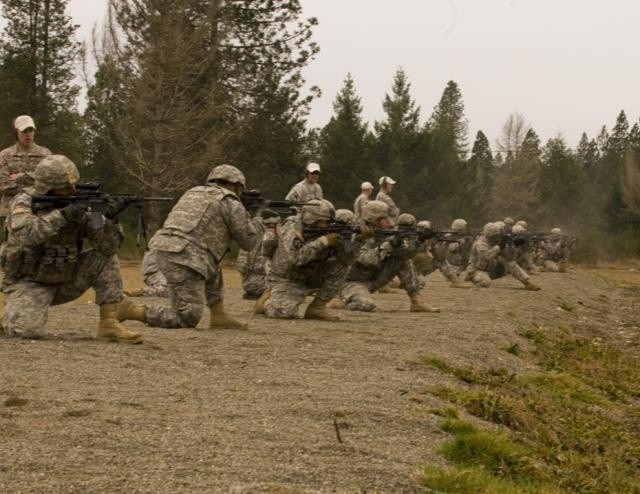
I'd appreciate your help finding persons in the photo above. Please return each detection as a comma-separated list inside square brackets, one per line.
[124, 246, 173, 297]
[375, 175, 401, 220]
[241, 199, 467, 320]
[284, 161, 324, 210]
[443, 217, 570, 290]
[0, 113, 54, 237]
[0, 154, 143, 345]
[119, 163, 266, 329]
[354, 181, 375, 217]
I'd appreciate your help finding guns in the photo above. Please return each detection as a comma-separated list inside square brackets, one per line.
[237, 189, 321, 219]
[399, 223, 584, 246]
[33, 182, 175, 219]
[303, 217, 407, 254]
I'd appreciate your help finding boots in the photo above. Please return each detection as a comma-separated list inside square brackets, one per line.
[524, 278, 541, 290]
[411, 291, 440, 312]
[97, 301, 143, 343]
[254, 288, 271, 314]
[124, 290, 145, 297]
[450, 275, 473, 288]
[117, 294, 146, 321]
[304, 296, 339, 321]
[379, 283, 397, 293]
[209, 300, 247, 330]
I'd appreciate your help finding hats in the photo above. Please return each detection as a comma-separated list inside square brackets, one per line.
[14, 114, 37, 131]
[361, 181, 374, 191]
[306, 163, 321, 173]
[379, 176, 396, 185]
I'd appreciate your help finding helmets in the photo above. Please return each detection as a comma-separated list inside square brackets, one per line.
[482, 217, 528, 236]
[550, 227, 562, 235]
[302, 197, 433, 234]
[450, 218, 468, 233]
[33, 154, 80, 194]
[206, 164, 245, 193]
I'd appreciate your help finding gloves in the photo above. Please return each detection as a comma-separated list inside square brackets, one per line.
[60, 203, 88, 228]
[355, 224, 373, 241]
[513, 237, 524, 246]
[105, 195, 125, 218]
[418, 232, 434, 242]
[324, 232, 342, 246]
[498, 238, 507, 250]
[390, 236, 403, 247]
